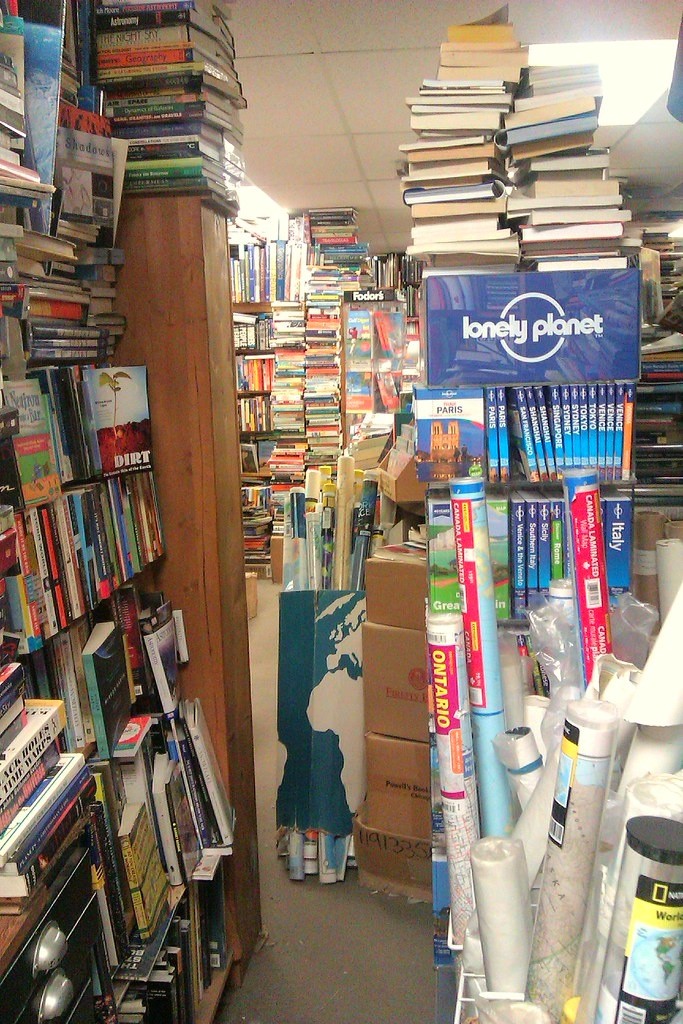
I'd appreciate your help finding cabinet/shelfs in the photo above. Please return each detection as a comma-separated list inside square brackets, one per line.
[110, 186, 263, 991]
[0, 847, 103, 1024]
[421, 478, 640, 625]
[232, 298, 345, 479]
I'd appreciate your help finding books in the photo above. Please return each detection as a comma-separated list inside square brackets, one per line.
[0, 3, 247, 1024]
[227, 2, 683, 625]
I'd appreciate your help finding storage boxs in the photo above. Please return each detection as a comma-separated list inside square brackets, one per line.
[352, 816, 433, 901]
[361, 621, 429, 742]
[356, 730, 434, 840]
[365, 557, 428, 630]
[270, 534, 284, 585]
[243, 572, 259, 620]
